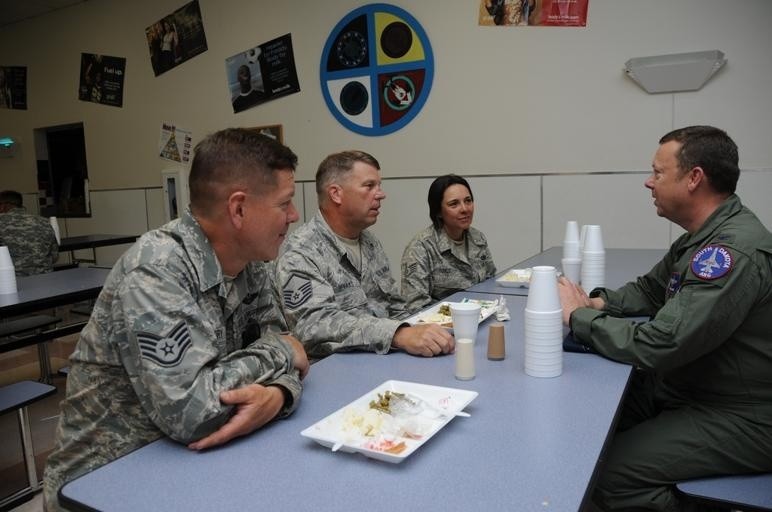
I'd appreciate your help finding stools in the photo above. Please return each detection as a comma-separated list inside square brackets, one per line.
[670, 472, 772, 511]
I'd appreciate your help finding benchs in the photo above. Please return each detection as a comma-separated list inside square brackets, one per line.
[1, 362, 69, 512]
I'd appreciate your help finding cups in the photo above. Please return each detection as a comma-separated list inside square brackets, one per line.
[449, 299, 482, 346]
[0, 244, 18, 295]
[560, 221, 607, 296]
[48, 216, 62, 246]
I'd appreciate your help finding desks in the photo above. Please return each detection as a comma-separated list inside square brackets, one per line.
[2, 229, 143, 384]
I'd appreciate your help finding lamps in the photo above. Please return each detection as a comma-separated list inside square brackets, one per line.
[623, 50, 728, 94]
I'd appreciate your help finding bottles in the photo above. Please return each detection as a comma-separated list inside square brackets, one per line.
[486, 324, 507, 361]
[523, 264, 564, 379]
[454, 336, 476, 381]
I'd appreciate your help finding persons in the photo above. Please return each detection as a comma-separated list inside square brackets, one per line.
[268, 150, 455, 366]
[400, 175, 496, 314]
[0, 191, 59, 277]
[558, 126, 772, 512]
[42, 129, 309, 512]
[160, 21, 179, 69]
[232, 65, 264, 113]
